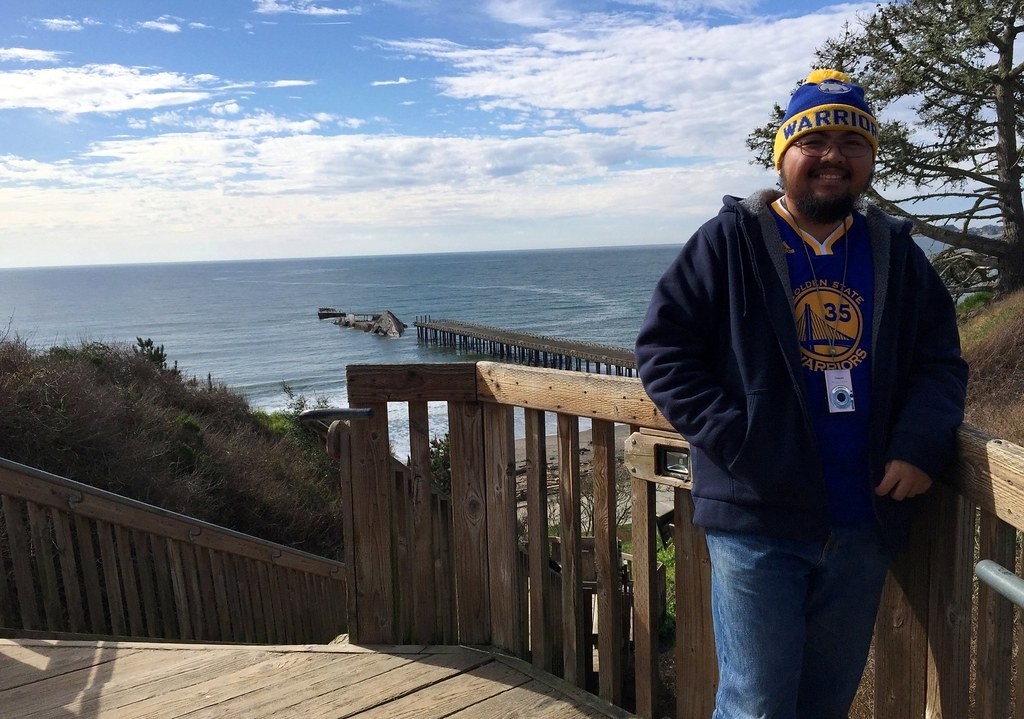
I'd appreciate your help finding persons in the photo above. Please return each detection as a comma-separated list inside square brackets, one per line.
[635, 67, 970, 719]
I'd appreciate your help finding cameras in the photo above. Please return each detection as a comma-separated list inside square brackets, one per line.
[824, 370, 855, 413]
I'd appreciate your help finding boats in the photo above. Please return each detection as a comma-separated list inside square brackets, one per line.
[317, 306, 347, 319]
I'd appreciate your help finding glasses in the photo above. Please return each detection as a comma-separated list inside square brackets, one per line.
[793, 132, 874, 159]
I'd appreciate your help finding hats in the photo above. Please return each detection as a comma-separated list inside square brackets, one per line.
[774, 67, 878, 172]
